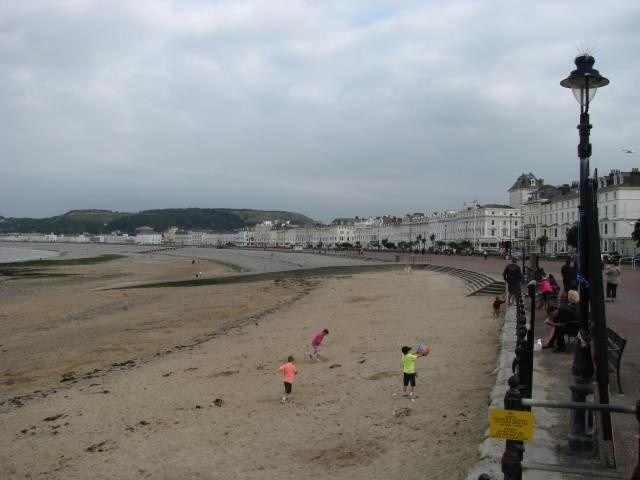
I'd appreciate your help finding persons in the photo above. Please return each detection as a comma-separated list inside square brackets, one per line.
[272, 355, 297, 404]
[400, 346, 422, 398]
[308, 328, 330, 363]
[441, 245, 621, 354]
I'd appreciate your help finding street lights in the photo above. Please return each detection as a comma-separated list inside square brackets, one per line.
[377, 229, 380, 252]
[509, 212, 513, 256]
[560, 51, 612, 449]
[444, 224, 447, 251]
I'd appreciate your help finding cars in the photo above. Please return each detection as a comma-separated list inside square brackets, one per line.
[601, 251, 622, 261]
[475, 249, 530, 260]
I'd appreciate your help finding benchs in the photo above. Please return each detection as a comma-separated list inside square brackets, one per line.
[544, 287, 563, 315]
[566, 320, 589, 352]
[594, 327, 628, 396]
